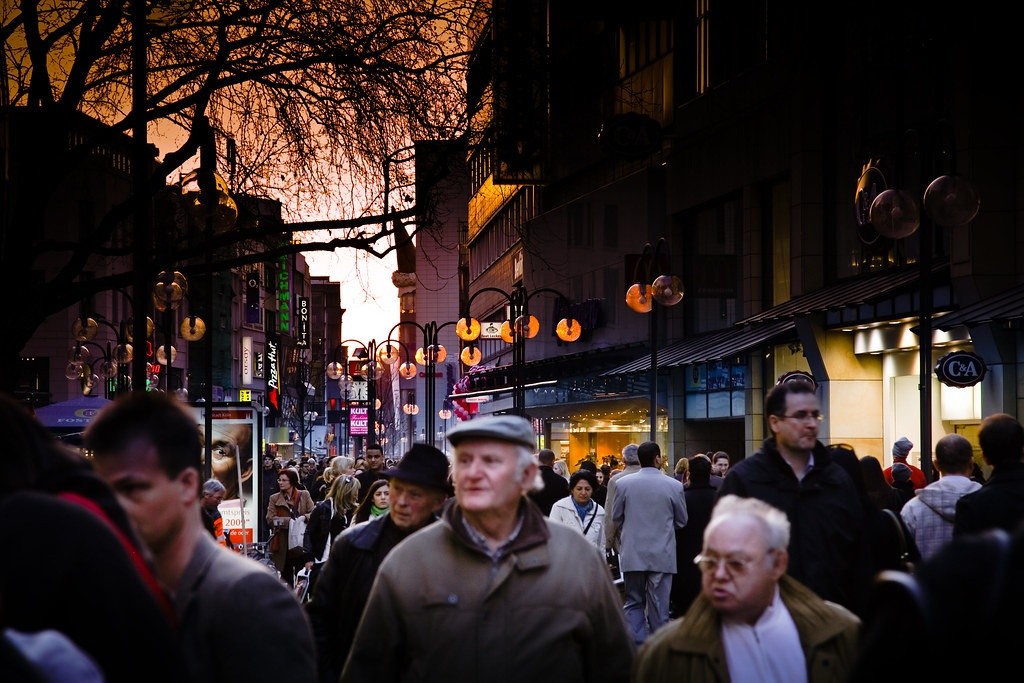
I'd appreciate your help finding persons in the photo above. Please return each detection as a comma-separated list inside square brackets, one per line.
[720, 382, 862, 612]
[1, 392, 319, 682]
[199, 425, 253, 500]
[826, 412, 1024, 683]
[265, 415, 861, 683]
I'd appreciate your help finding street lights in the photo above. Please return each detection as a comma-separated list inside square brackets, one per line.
[61, 286, 206, 399]
[853, 151, 983, 488]
[150, 268, 189, 397]
[624, 235, 685, 444]
[463, 278, 583, 420]
[379, 319, 482, 447]
[327, 338, 417, 449]
[173, 167, 239, 485]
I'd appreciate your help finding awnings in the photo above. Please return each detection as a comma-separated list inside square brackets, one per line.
[602, 319, 796, 377]
[914, 286, 1024, 335]
[735, 258, 948, 325]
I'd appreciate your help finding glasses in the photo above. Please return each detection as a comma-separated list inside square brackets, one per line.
[277, 478, 290, 483]
[344, 475, 352, 485]
[777, 412, 825, 421]
[694, 553, 772, 577]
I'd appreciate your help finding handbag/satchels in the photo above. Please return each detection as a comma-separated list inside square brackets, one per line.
[607, 546, 624, 584]
[288, 519, 307, 552]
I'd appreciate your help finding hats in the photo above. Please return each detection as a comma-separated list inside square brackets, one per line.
[891, 463, 912, 483]
[447, 415, 536, 448]
[892, 437, 913, 458]
[379, 443, 454, 490]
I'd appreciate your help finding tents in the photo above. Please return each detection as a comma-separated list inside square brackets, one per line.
[34, 395, 112, 429]
